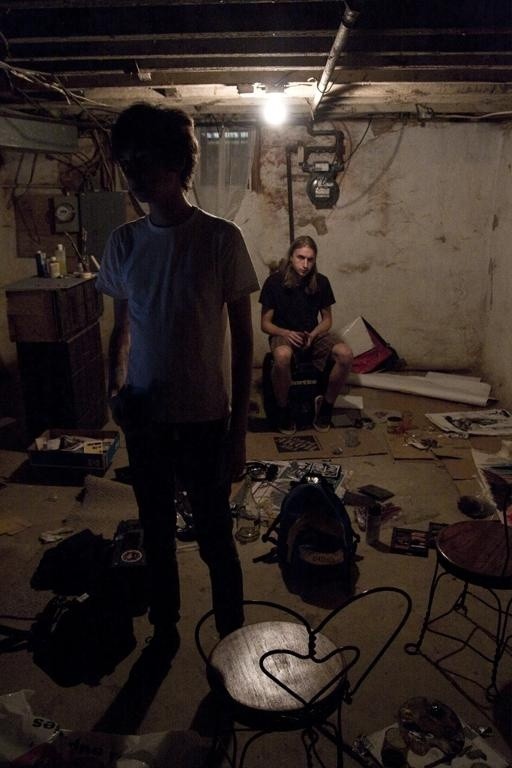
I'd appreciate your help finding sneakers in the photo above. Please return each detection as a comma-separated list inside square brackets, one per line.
[313, 395, 330, 433]
[274, 400, 297, 435]
[127, 629, 180, 682]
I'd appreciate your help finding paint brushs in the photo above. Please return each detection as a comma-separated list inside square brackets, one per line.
[63, 227, 88, 273]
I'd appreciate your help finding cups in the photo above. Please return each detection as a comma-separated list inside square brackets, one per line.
[381, 728, 413, 768]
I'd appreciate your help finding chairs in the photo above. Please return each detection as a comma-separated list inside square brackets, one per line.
[195, 589, 414, 765]
[403, 465, 512, 700]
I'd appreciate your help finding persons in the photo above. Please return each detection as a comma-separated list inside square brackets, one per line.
[258, 235, 353, 436]
[90, 103, 260, 660]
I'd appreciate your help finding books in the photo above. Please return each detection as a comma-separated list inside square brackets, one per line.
[60, 439, 103, 454]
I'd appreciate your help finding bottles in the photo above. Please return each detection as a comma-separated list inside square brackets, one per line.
[237, 475, 260, 540]
[35, 244, 68, 279]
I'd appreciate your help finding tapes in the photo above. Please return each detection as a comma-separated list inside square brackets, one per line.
[386, 417, 402, 427]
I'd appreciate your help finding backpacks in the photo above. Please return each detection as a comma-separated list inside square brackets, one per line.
[262, 472, 360, 598]
